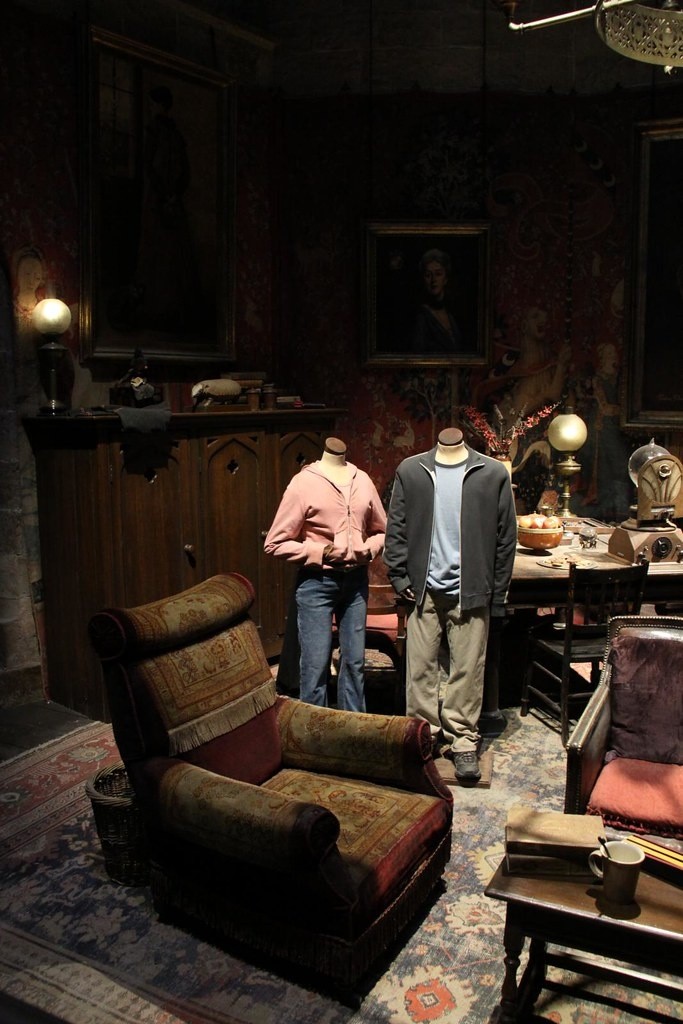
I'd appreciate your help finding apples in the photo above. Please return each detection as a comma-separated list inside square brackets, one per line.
[518, 513, 563, 529]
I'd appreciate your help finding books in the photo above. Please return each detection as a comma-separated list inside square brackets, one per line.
[503, 806, 607, 878]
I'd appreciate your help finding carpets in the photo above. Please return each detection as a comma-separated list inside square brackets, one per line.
[0, 682, 683, 1024]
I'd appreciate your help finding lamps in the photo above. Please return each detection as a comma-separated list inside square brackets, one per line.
[31, 298, 71, 415]
[546, 413, 587, 519]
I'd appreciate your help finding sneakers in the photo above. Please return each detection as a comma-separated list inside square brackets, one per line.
[453, 750, 481, 779]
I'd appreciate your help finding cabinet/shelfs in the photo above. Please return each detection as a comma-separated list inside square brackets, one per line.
[26, 407, 348, 727]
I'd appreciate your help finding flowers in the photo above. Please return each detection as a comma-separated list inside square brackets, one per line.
[456, 397, 562, 461]
[393, 371, 452, 450]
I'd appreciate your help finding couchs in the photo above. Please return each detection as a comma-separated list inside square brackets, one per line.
[87, 572, 453, 990]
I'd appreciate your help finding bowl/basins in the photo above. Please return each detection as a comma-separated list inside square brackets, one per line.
[516, 526, 563, 550]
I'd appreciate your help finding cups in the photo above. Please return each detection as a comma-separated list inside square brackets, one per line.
[588, 840, 645, 904]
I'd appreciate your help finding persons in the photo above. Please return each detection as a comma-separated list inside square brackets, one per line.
[383, 428, 519, 779]
[263, 437, 391, 713]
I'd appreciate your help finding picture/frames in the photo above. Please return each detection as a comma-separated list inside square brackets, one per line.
[77, 24, 241, 365]
[360, 215, 496, 372]
[617, 119, 683, 435]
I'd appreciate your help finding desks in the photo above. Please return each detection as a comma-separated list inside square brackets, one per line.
[485, 842, 683, 1024]
[506, 511, 683, 635]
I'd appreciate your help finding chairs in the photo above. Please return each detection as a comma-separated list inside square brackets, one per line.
[327, 574, 406, 691]
[520, 565, 651, 744]
[565, 616, 683, 839]
